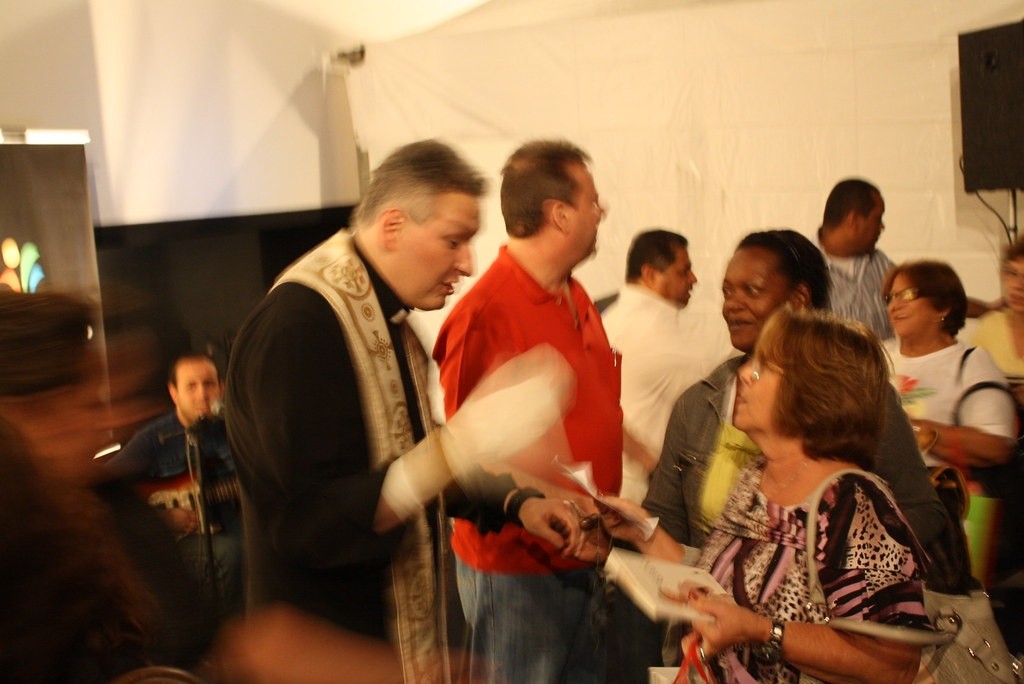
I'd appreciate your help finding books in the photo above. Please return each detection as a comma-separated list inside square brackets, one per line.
[606, 548, 738, 621]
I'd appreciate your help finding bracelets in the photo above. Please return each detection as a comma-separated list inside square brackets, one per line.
[506, 485, 545, 528]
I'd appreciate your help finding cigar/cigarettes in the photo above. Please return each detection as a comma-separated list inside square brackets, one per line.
[561, 512, 599, 537]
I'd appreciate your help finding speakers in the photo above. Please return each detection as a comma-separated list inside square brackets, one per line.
[959, 19, 1024, 190]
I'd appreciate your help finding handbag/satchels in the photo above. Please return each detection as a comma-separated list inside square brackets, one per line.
[939, 346, 1023, 590]
[798, 467, 1023, 684]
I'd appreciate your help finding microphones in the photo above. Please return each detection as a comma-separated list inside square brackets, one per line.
[209, 401, 226, 420]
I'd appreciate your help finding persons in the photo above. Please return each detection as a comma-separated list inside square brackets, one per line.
[1, 140, 1024, 684]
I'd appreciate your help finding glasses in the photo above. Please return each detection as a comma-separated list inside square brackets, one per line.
[728, 349, 791, 383]
[883, 286, 940, 311]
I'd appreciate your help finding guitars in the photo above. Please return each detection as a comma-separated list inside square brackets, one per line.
[130, 471, 241, 542]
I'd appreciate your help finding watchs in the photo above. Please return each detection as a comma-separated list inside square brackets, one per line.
[759, 619, 783, 666]
